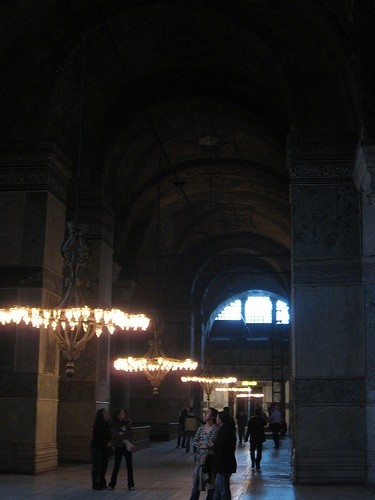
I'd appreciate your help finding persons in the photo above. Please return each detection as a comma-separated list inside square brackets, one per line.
[90, 408, 136, 491]
[200, 411, 237, 500]
[176, 401, 287, 454]
[244, 407, 267, 470]
[188, 407, 221, 500]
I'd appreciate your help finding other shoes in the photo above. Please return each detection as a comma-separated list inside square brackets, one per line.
[128, 486, 136, 491]
[176, 444, 180, 448]
[252, 462, 254, 468]
[93, 485, 108, 490]
[182, 442, 186, 448]
[109, 482, 117, 489]
[256, 466, 260, 469]
[273, 447, 279, 449]
[239, 441, 242, 443]
[244, 438, 248, 441]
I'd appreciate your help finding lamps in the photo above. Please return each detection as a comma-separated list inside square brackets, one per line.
[0, 227, 150, 377]
[180, 373, 238, 409]
[112, 311, 197, 396]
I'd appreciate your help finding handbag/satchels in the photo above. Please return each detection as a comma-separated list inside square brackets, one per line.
[125, 442, 137, 452]
